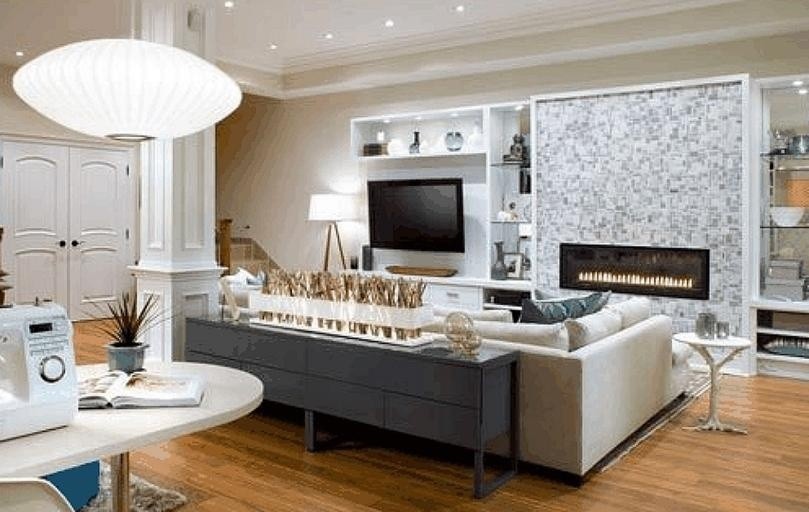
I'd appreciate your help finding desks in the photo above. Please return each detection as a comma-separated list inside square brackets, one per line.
[184, 309, 521, 499]
[0, 357, 264, 512]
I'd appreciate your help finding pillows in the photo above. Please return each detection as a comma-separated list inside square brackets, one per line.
[423, 288, 651, 352]
[220, 288, 248, 307]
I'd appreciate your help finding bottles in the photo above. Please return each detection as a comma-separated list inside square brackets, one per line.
[775, 137, 787, 154]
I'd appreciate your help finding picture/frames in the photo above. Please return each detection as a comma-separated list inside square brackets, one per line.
[503, 253, 523, 279]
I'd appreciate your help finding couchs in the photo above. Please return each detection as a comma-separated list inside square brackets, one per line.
[219, 283, 693, 488]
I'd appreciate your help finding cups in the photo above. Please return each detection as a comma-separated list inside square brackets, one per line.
[794, 134, 809, 154]
[696, 312, 730, 340]
[351, 256, 357, 269]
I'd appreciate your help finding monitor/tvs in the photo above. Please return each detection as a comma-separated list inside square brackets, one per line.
[368, 178, 464, 252]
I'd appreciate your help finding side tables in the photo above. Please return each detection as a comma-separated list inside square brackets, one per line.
[673, 332, 751, 434]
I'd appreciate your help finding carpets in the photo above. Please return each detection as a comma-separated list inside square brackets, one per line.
[79, 460, 188, 512]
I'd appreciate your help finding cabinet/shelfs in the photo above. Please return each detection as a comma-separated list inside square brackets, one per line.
[350, 101, 531, 313]
[747, 74, 809, 382]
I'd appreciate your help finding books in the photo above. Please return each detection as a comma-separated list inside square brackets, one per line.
[77, 369, 205, 409]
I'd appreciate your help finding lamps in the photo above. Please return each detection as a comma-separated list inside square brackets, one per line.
[10, 0, 243, 141]
[307, 193, 356, 272]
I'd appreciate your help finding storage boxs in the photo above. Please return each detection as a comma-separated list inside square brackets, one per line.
[765, 259, 805, 301]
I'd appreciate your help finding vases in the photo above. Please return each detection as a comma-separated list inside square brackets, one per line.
[409, 130, 421, 154]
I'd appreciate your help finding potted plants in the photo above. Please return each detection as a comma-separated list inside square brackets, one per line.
[77, 291, 184, 372]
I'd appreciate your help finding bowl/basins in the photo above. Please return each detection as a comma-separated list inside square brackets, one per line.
[770, 206, 805, 226]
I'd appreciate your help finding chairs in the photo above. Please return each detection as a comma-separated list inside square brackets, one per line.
[0, 478, 77, 512]
